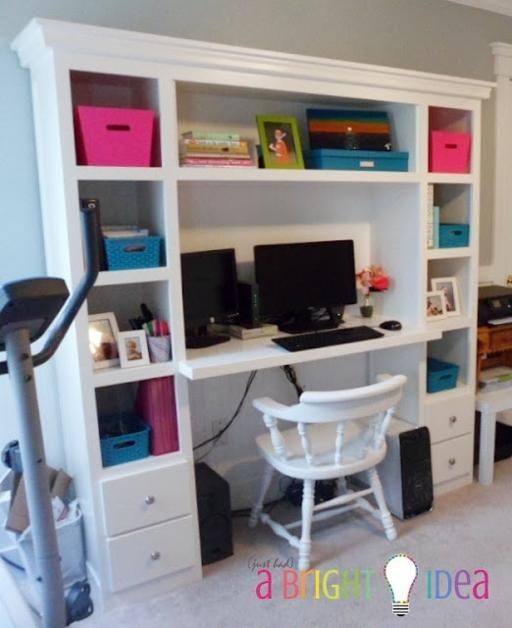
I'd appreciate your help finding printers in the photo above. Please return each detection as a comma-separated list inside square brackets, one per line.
[478, 285, 512, 327]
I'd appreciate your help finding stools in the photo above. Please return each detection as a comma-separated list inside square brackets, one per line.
[474, 381, 511, 486]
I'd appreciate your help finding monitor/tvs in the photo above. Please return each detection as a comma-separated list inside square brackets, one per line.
[181, 248, 240, 348]
[254, 240, 356, 334]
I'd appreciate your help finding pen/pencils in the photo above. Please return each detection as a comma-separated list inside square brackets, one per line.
[130, 303, 168, 335]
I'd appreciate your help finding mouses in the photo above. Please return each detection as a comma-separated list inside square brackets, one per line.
[380, 320, 401, 330]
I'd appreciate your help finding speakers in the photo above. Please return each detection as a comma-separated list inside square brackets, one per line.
[195, 463, 234, 565]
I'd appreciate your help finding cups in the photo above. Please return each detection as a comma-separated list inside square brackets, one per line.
[147, 335, 172, 363]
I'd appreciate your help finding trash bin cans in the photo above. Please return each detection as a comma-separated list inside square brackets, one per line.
[2, 508, 88, 622]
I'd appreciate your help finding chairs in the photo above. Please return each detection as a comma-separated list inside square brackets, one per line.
[249, 372, 409, 570]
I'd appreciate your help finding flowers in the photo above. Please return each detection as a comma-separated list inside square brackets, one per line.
[356, 264, 395, 306]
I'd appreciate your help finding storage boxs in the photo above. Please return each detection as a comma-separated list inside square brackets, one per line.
[302, 148, 409, 172]
[430, 131, 470, 175]
[99, 411, 151, 470]
[439, 222, 469, 250]
[75, 105, 154, 167]
[427, 357, 459, 394]
[101, 233, 163, 272]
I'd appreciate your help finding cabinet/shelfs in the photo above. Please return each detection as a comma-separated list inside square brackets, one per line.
[11, 15, 498, 611]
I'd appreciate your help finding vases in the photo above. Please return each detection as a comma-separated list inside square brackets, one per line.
[360, 305, 373, 318]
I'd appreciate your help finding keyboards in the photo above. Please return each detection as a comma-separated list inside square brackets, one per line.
[273, 326, 383, 352]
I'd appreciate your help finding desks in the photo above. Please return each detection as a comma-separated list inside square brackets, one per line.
[179, 309, 440, 382]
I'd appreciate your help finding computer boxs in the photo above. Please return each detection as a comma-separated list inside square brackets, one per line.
[348, 417, 434, 521]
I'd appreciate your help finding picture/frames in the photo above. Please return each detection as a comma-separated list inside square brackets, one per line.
[87, 312, 122, 369]
[426, 290, 448, 321]
[431, 277, 459, 317]
[256, 116, 305, 170]
[116, 330, 149, 368]
